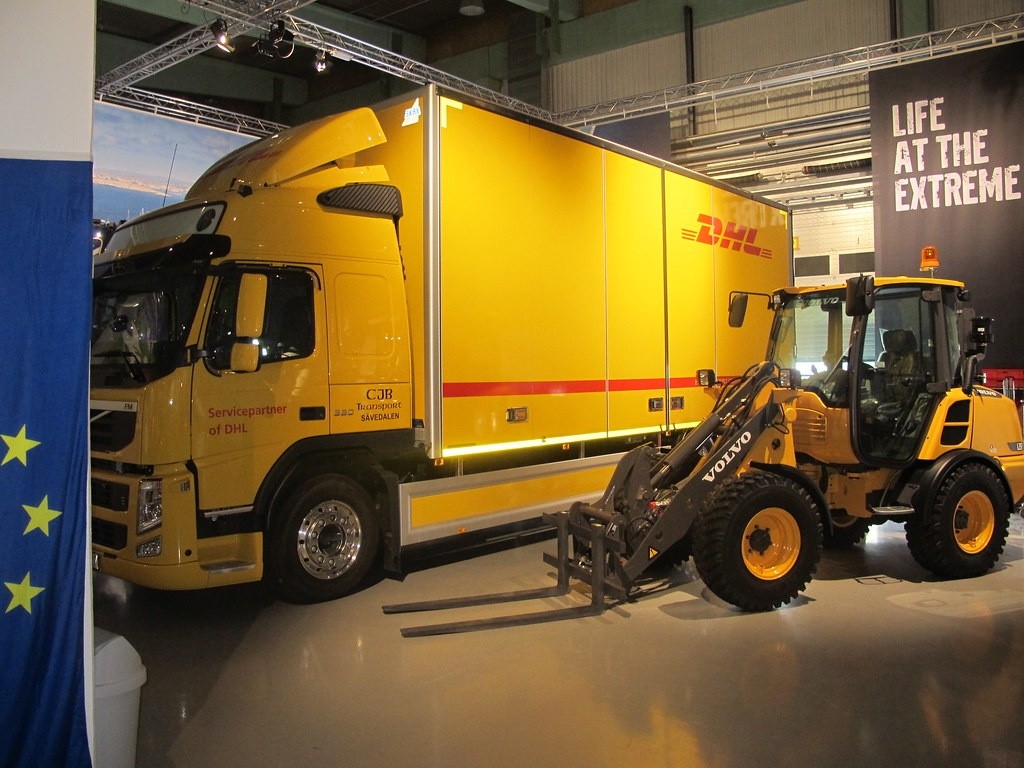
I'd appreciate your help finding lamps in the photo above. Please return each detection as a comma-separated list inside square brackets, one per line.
[210, 19, 236, 54]
[314, 48, 327, 72]
[250, 18, 295, 60]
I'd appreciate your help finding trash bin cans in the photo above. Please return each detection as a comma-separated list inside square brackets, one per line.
[94, 627, 147, 768]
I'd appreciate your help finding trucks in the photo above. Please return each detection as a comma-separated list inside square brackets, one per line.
[90, 82, 794, 604]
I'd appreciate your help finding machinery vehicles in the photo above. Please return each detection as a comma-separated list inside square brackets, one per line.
[382, 244, 1024, 635]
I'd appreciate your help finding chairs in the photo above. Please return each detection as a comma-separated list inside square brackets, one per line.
[859, 328, 923, 425]
